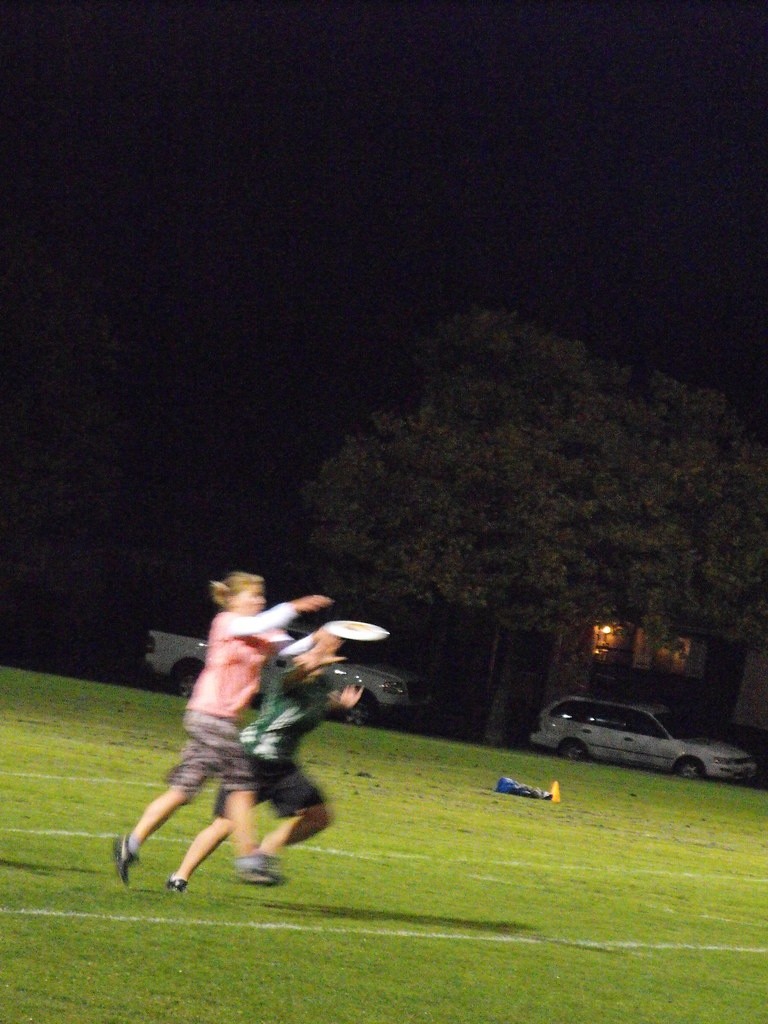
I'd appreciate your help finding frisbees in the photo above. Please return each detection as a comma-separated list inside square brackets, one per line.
[324, 620, 388, 642]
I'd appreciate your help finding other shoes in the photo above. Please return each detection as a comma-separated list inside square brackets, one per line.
[112, 834, 138, 887]
[167, 872, 187, 893]
[236, 855, 279, 885]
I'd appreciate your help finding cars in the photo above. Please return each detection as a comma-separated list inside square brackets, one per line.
[527, 696, 759, 785]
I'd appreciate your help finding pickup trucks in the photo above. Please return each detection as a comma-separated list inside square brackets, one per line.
[145, 620, 415, 730]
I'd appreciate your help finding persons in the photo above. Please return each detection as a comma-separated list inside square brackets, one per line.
[112, 571, 341, 885]
[166, 635, 365, 894]
